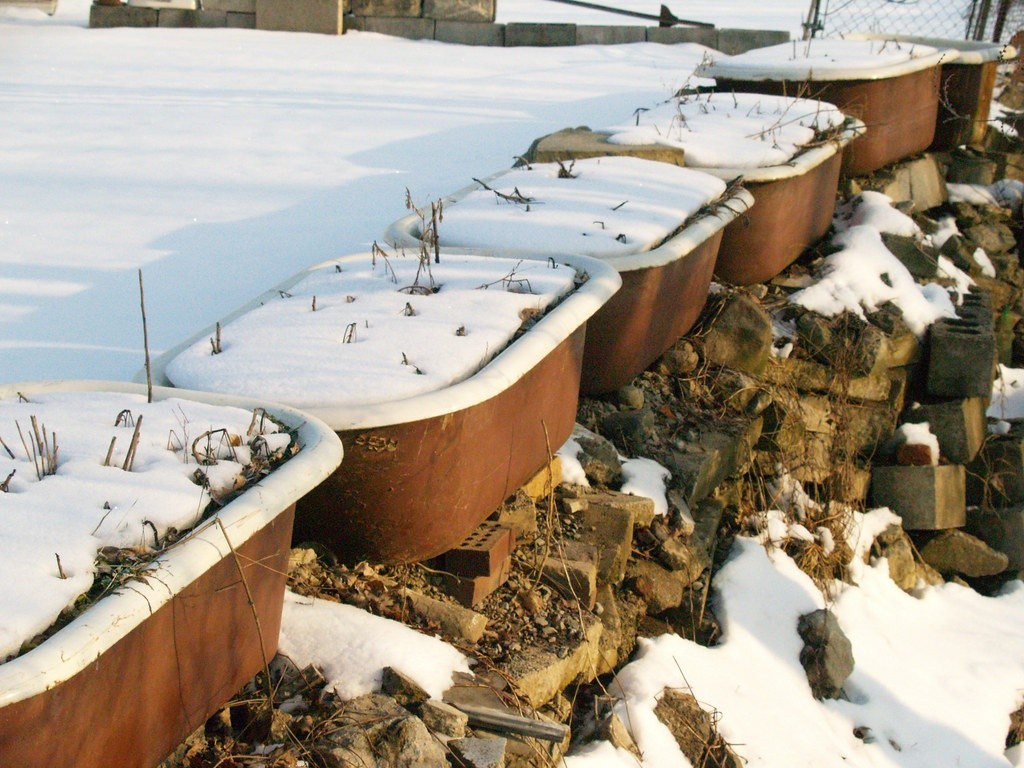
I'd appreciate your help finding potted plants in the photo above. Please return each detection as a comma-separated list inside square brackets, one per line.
[571, 67, 888, 288]
[688, 26, 1013, 178]
[174, 185, 624, 571]
[383, 147, 764, 399]
[0, 266, 348, 768]
